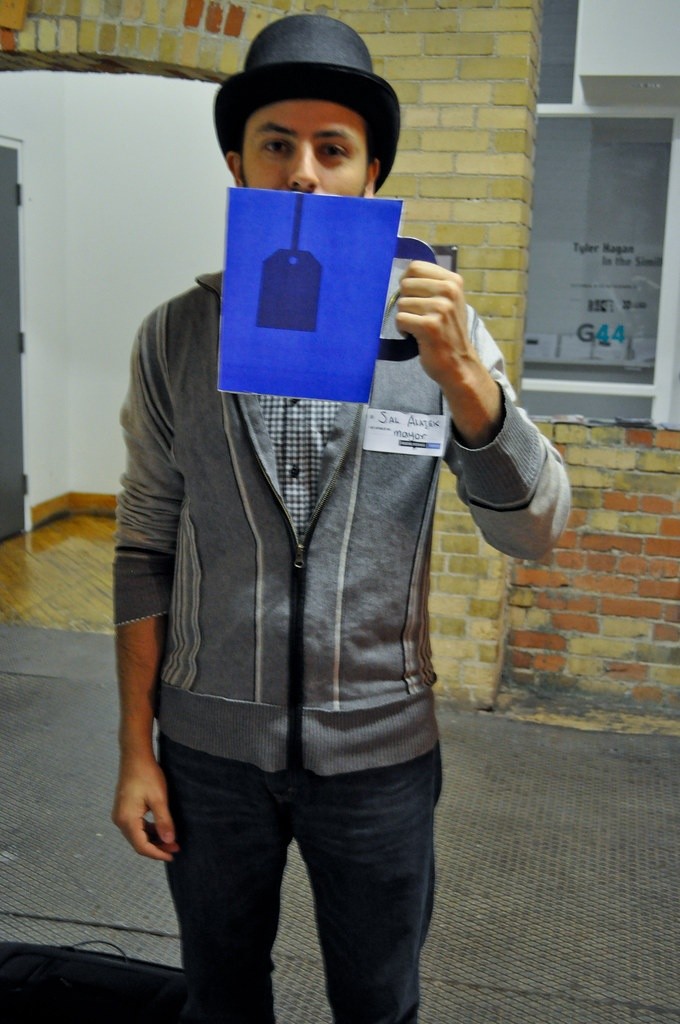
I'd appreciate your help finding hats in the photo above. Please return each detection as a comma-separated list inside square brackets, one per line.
[211, 13, 402, 193]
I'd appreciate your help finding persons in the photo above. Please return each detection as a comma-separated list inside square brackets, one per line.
[111, 13, 571, 1023]
